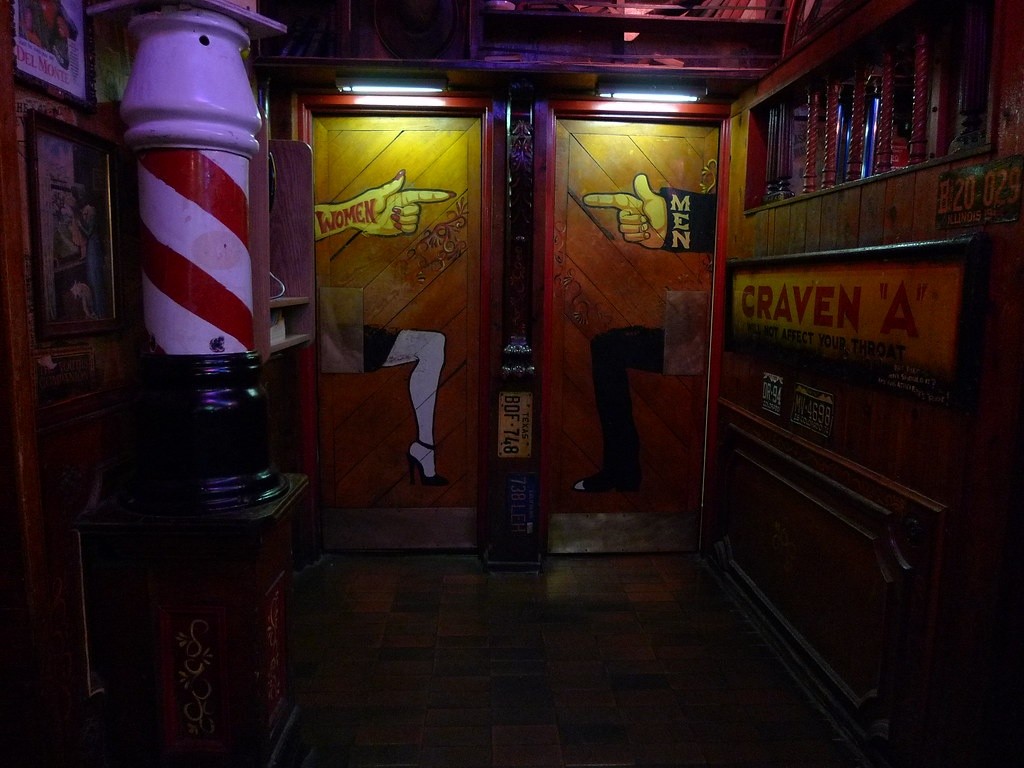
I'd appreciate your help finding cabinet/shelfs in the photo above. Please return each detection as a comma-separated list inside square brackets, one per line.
[714, 0, 1024, 768]
[76, 473, 312, 768]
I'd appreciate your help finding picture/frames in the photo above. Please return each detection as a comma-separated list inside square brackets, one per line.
[8, 0, 98, 113]
[23, 107, 128, 340]
[36, 346, 97, 404]
